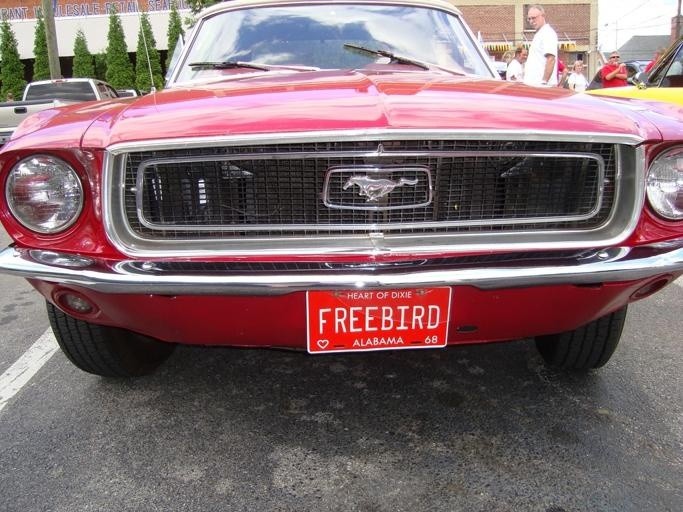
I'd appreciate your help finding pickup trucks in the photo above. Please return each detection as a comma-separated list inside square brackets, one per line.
[0, 76, 118, 149]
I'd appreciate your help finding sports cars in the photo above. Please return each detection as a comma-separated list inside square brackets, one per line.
[0, 2, 682, 375]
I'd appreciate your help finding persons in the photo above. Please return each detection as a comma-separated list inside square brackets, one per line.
[600, 51, 628, 88]
[524, 4, 558, 88]
[566, 59, 588, 93]
[644, 46, 667, 73]
[504, 47, 528, 82]
[556, 60, 568, 88]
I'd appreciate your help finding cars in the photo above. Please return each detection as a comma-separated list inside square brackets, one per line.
[581, 35, 682, 108]
[587, 61, 681, 91]
